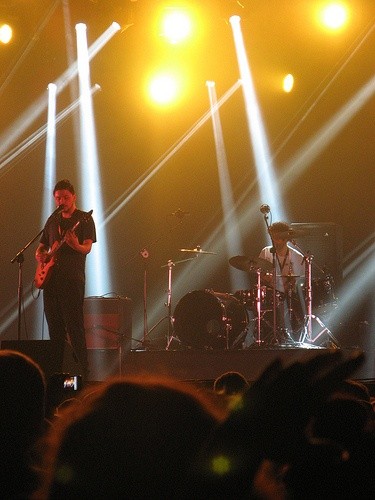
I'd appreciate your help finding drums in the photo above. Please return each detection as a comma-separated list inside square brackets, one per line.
[173, 289, 250, 351]
[240, 288, 279, 312]
[300, 274, 343, 315]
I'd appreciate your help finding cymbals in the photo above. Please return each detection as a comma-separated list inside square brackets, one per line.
[177, 249, 217, 255]
[229, 254, 275, 274]
[275, 274, 301, 278]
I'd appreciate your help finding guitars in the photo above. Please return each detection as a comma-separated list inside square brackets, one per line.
[33, 209, 94, 290]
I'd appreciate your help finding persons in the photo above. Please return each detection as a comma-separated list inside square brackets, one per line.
[259, 222, 305, 291]
[35, 179, 97, 379]
[0, 350, 375, 500]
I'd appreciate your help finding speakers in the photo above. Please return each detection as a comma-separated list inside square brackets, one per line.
[65, 298, 133, 349]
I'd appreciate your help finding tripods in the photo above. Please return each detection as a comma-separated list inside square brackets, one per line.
[144, 212, 342, 351]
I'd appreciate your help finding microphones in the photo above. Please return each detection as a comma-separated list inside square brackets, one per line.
[48, 204, 64, 222]
